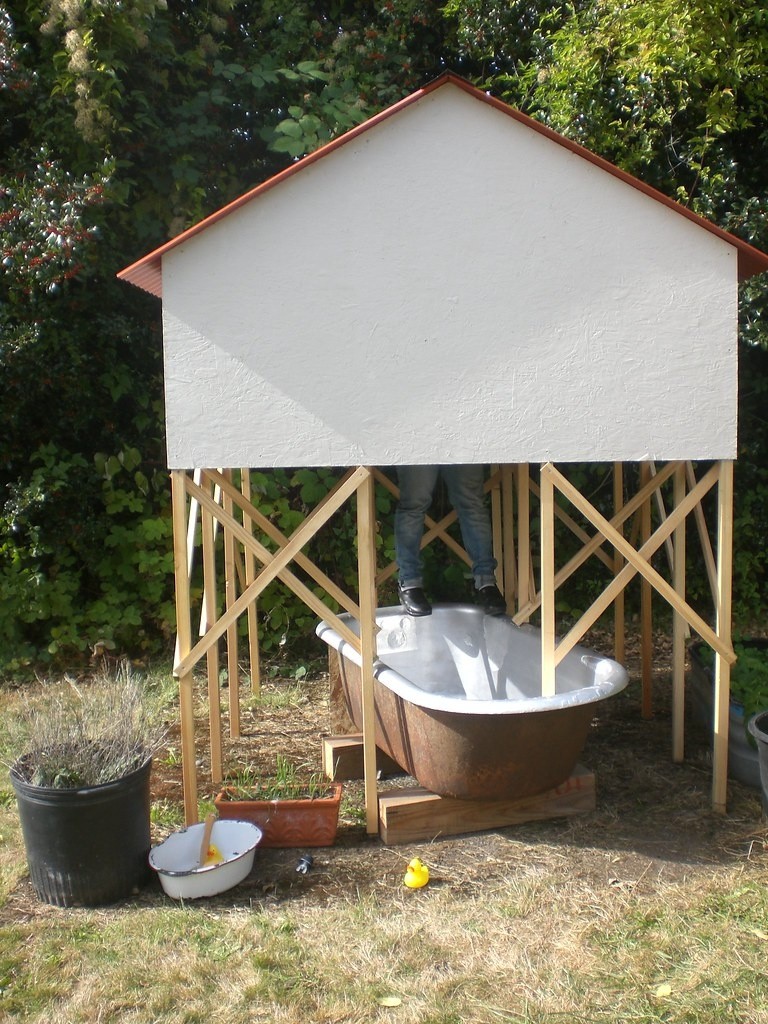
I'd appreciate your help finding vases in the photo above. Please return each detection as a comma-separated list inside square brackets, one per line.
[746, 711, 768, 818]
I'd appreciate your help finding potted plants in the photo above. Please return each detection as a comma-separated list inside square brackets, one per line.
[0, 648, 181, 909]
[688, 637, 768, 787]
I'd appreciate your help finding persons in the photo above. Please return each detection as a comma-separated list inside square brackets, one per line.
[395, 464, 507, 616]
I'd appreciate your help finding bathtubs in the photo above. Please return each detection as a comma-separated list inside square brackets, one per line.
[315, 601, 630, 803]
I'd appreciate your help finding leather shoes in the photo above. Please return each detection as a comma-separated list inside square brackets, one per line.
[476, 584, 506, 615]
[397, 585, 432, 617]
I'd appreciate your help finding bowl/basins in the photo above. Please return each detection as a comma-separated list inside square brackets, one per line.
[148, 819, 263, 901]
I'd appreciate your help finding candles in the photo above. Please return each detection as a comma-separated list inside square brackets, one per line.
[211, 753, 343, 848]
